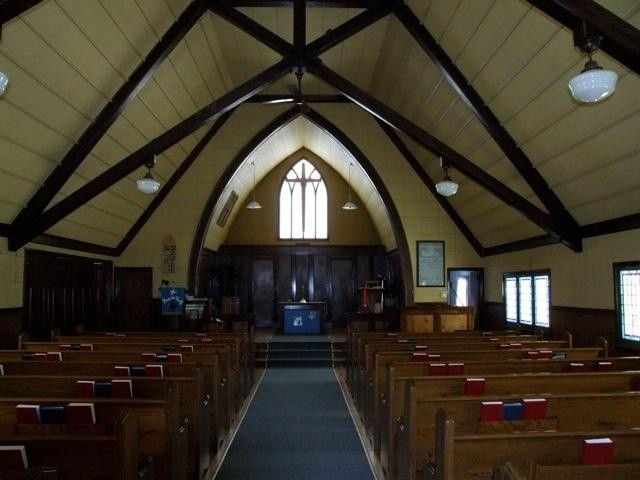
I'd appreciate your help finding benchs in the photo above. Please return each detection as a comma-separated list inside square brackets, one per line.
[346, 324, 640, 479]
[0, 318, 257, 480]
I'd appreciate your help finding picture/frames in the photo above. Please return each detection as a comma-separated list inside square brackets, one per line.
[416, 240, 446, 288]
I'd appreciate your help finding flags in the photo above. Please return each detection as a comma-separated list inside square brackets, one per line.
[160, 286, 187, 315]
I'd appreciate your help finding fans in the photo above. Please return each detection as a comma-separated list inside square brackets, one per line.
[241, 65, 356, 105]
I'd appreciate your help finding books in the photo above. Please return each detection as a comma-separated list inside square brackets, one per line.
[481, 398, 548, 420]
[386, 332, 442, 358]
[599, 361, 612, 373]
[464, 378, 486, 396]
[14, 400, 96, 426]
[569, 362, 585, 373]
[21, 351, 63, 361]
[448, 362, 465, 376]
[76, 377, 136, 401]
[0, 363, 6, 376]
[581, 437, 616, 466]
[483, 332, 566, 359]
[0, 441, 31, 473]
[106, 332, 213, 378]
[428, 363, 447, 376]
[60, 344, 94, 351]
[196, 334, 206, 340]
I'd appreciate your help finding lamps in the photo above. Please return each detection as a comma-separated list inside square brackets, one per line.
[435, 155, 460, 198]
[245, 161, 262, 210]
[566, 20, 620, 102]
[342, 162, 360, 212]
[136, 153, 161, 194]
